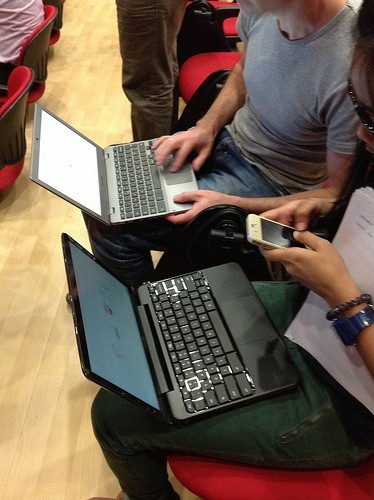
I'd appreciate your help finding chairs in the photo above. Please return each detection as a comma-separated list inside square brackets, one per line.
[0, 65, 35, 194]
[172, 0, 242, 131]
[169, 456, 374, 499]
[0, 4, 58, 104]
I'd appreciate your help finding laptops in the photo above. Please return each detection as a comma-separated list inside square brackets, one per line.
[30, 103, 199, 226]
[61, 232, 302, 430]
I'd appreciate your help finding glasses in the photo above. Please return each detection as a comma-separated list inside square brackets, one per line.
[346, 79, 374, 136]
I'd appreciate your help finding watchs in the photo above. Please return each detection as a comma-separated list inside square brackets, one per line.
[333, 305, 374, 347]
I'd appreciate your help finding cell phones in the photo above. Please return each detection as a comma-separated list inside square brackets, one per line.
[246, 214, 314, 251]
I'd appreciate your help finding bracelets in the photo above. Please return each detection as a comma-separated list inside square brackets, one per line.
[325, 293, 371, 321]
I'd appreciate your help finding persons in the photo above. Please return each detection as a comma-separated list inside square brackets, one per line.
[66, 0, 362, 305]
[108, 0, 194, 147]
[91, 0, 374, 500]
[0, 0, 45, 97]
